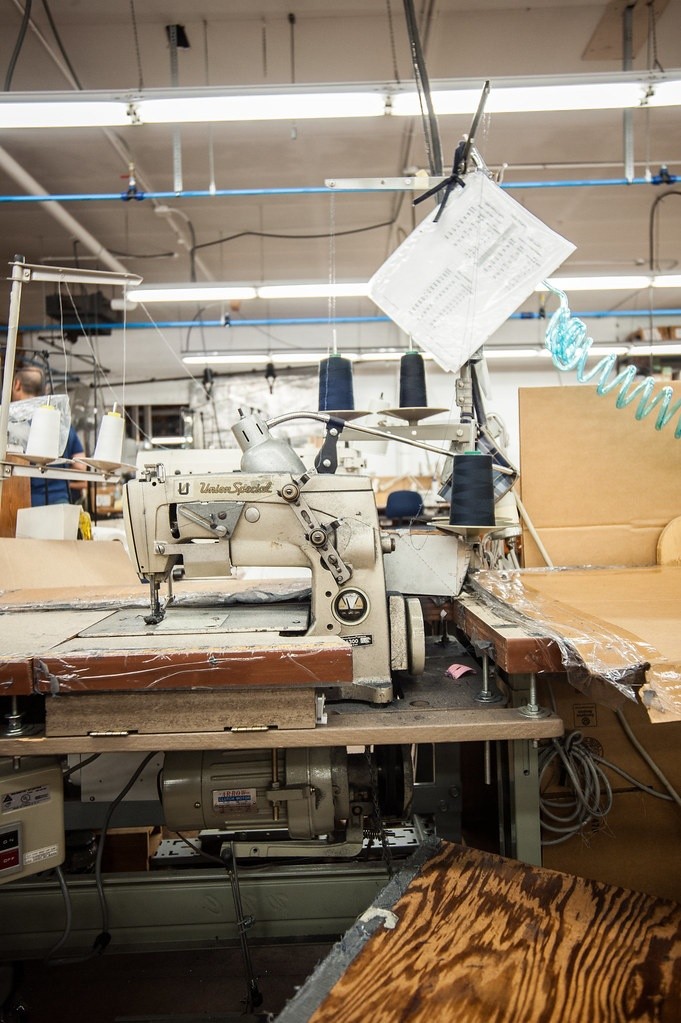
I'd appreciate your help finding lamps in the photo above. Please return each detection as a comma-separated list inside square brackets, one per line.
[230, 414, 519, 480]
[0, 0, 681, 129]
[182, 301, 681, 364]
[126, 194, 681, 302]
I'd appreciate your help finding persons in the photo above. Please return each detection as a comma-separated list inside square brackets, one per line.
[12, 367, 87, 508]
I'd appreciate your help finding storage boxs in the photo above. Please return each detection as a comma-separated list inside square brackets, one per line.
[488, 383, 681, 902]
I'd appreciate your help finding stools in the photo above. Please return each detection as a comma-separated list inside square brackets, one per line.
[270, 838, 681, 1023]
